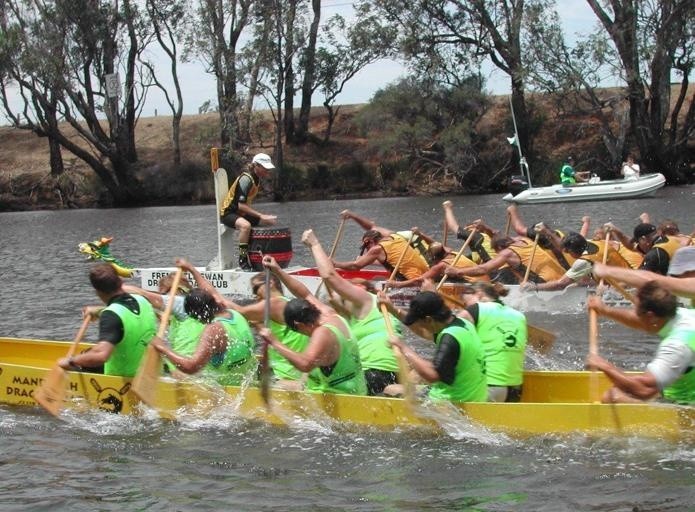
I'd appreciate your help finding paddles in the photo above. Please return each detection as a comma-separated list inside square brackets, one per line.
[380, 291, 417, 405]
[130, 266, 183, 407]
[437, 290, 558, 357]
[32, 313, 92, 417]
[259, 257, 272, 405]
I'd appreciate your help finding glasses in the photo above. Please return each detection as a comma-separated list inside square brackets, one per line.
[252, 281, 269, 292]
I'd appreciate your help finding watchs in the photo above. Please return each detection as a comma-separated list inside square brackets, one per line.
[66, 353, 72, 366]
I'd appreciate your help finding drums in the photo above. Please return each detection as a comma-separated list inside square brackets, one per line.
[246, 223, 294, 271]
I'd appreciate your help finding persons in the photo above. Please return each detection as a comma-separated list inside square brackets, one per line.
[444, 218, 567, 285]
[443, 201, 518, 285]
[521, 232, 634, 291]
[301, 229, 403, 398]
[150, 258, 257, 387]
[579, 216, 645, 271]
[640, 213, 695, 248]
[596, 246, 695, 310]
[604, 222, 684, 277]
[220, 153, 277, 272]
[395, 230, 442, 284]
[119, 273, 212, 378]
[376, 290, 489, 402]
[386, 227, 491, 283]
[506, 204, 571, 271]
[328, 209, 430, 288]
[222, 271, 309, 391]
[622, 154, 640, 180]
[584, 281, 695, 407]
[421, 277, 528, 403]
[260, 255, 368, 396]
[561, 156, 591, 186]
[58, 263, 165, 376]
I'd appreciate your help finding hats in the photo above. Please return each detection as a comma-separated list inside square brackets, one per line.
[402, 290, 443, 326]
[358, 231, 381, 251]
[629, 223, 656, 242]
[251, 153, 277, 169]
[528, 224, 555, 245]
[558, 231, 598, 255]
[668, 246, 695, 276]
[567, 156, 573, 160]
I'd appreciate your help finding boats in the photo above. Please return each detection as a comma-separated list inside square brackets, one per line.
[76, 148, 695, 323]
[502, 173, 666, 204]
[0, 338, 695, 446]
